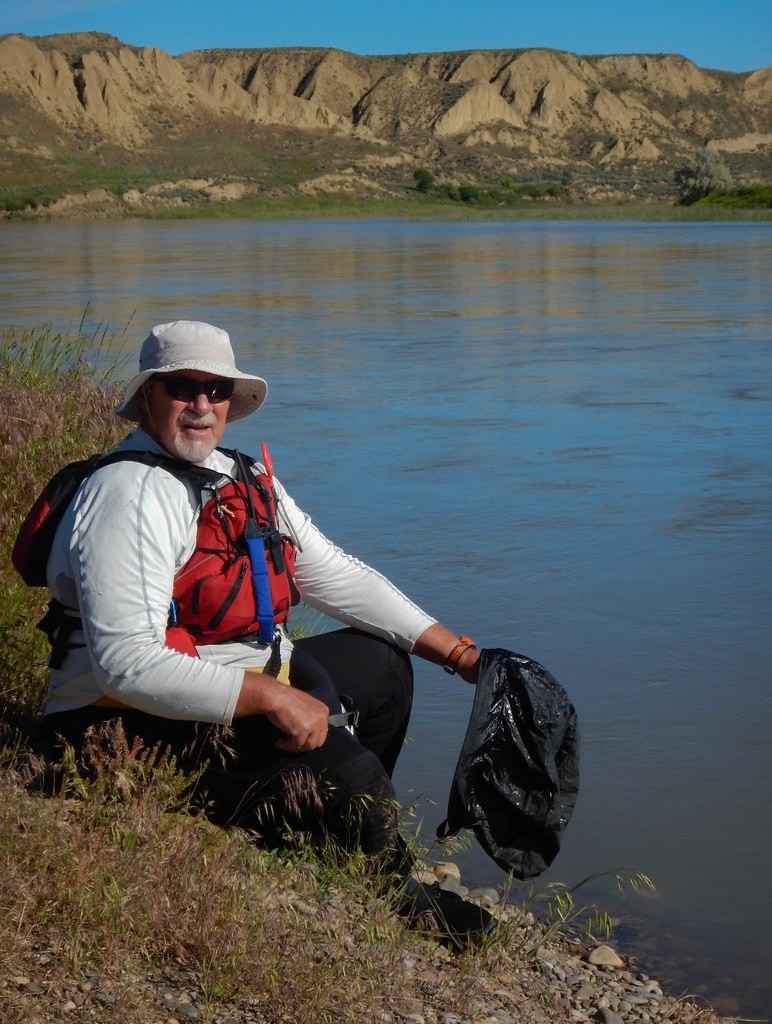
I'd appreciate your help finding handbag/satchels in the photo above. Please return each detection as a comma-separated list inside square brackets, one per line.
[434, 648, 579, 881]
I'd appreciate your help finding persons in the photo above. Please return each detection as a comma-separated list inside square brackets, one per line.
[41, 320, 499, 939]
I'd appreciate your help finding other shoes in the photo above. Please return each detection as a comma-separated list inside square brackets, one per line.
[404, 882, 496, 943]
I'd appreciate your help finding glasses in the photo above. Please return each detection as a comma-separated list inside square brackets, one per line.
[149, 375, 234, 404]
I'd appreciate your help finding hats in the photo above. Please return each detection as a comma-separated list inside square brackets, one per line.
[115, 321, 266, 424]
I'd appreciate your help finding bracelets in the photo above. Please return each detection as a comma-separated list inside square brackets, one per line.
[443, 636, 477, 676]
